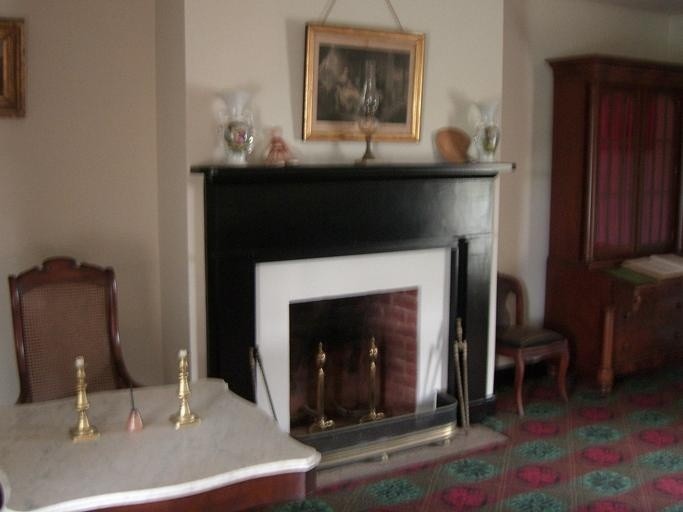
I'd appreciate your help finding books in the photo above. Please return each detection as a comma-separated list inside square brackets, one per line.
[623, 252, 683, 280]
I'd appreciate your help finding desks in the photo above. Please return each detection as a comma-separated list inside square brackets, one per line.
[0, 377, 322, 512]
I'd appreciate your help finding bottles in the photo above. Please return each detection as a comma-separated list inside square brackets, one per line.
[318, 46, 403, 123]
[473, 101, 501, 163]
[357, 58, 380, 161]
[217, 107, 260, 166]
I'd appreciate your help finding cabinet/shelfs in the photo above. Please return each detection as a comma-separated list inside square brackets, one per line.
[544, 53, 683, 398]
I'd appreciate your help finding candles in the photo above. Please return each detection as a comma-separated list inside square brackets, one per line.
[178, 349, 187, 358]
[75, 355, 85, 366]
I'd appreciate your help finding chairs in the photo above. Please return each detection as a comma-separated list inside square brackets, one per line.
[8, 255, 145, 404]
[496, 272, 569, 416]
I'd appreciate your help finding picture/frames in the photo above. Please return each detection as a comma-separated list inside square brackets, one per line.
[0, 19, 25, 119]
[302, 23, 425, 145]
